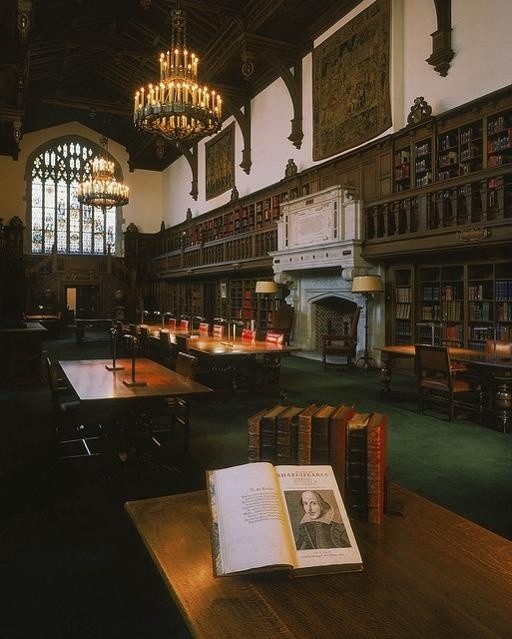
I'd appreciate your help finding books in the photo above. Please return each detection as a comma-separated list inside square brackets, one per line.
[139, 182, 307, 340]
[204, 459, 366, 581]
[394, 110, 512, 353]
[246, 403, 388, 527]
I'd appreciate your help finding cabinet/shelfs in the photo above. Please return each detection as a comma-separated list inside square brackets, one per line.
[389, 106, 512, 349]
[137, 182, 310, 344]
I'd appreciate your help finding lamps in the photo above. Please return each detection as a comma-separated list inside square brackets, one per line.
[133, 0, 222, 148]
[78, 113, 130, 214]
[352, 275, 384, 367]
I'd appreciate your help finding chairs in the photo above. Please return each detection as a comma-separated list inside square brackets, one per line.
[415, 339, 512, 425]
[46, 321, 213, 462]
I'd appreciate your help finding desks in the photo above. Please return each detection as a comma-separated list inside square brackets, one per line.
[125, 324, 302, 394]
[373, 345, 512, 434]
[124, 481, 512, 639]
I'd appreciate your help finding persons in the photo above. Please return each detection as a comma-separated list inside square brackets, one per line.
[296, 489, 353, 550]
